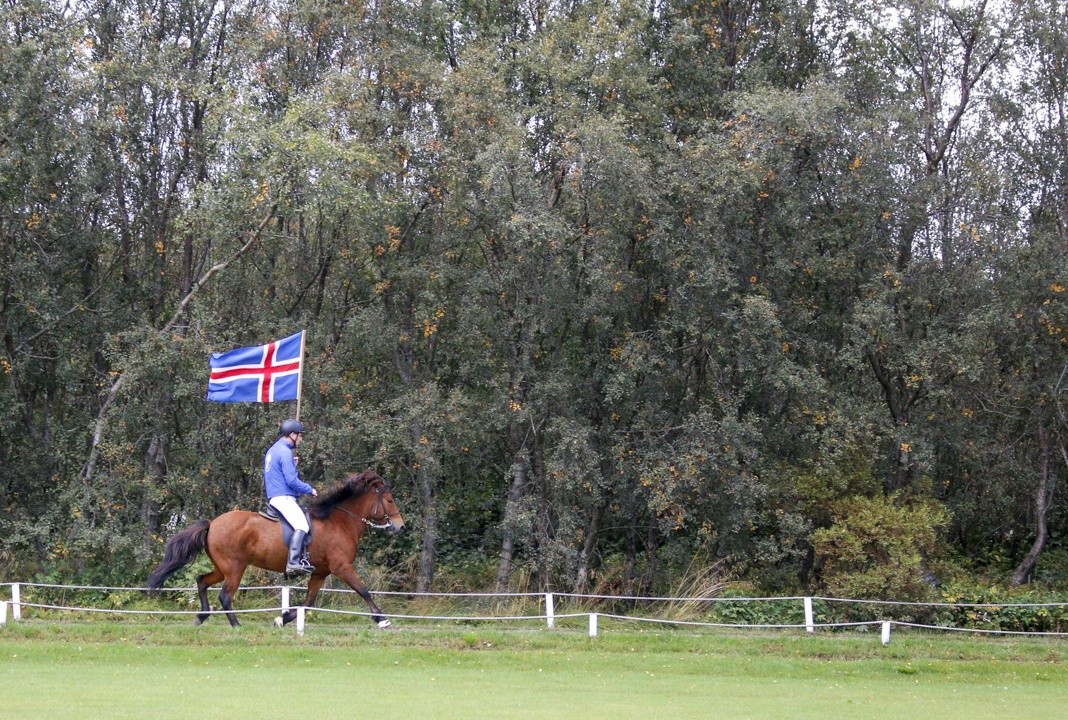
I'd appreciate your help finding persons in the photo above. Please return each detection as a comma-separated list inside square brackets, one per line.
[264, 419, 318, 574]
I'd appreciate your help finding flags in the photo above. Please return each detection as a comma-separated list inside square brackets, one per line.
[207, 329, 306, 402]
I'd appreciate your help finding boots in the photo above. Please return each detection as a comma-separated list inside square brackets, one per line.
[286, 529, 315, 574]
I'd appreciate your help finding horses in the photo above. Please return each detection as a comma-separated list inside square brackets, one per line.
[141, 465, 409, 631]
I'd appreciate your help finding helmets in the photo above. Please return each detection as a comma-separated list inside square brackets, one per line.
[279, 419, 306, 436]
